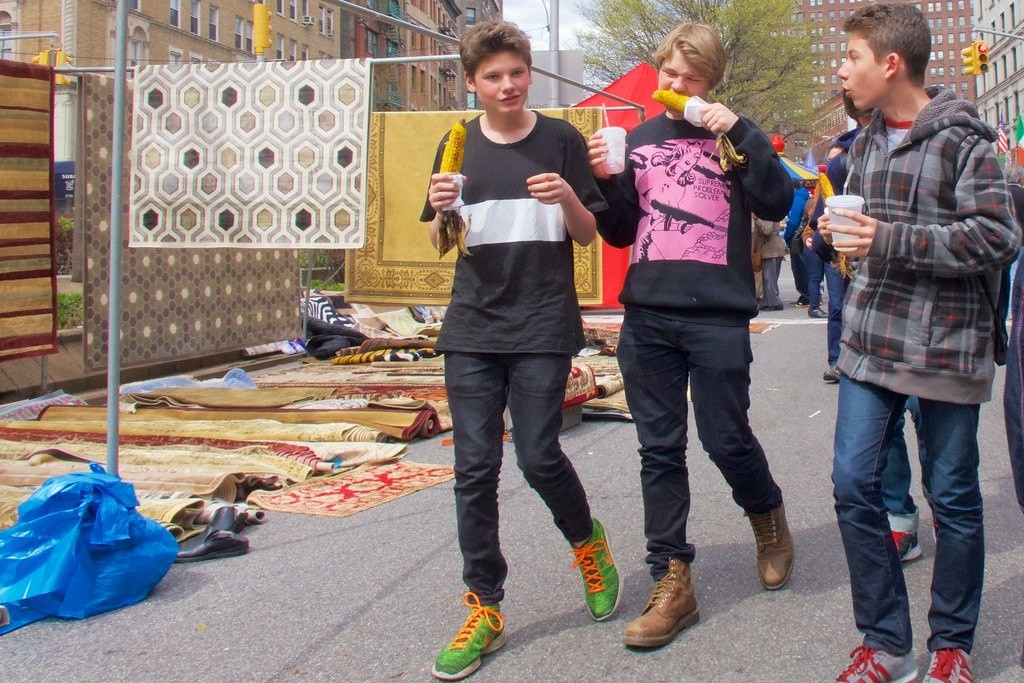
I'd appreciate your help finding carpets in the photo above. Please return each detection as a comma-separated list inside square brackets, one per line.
[749, 321, 781, 334]
[0, 60, 633, 542]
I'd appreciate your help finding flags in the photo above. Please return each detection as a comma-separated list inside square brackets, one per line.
[1016, 111, 1024, 150]
[997, 113, 1008, 152]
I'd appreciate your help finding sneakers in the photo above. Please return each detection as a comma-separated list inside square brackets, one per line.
[891, 531, 922, 562]
[836, 646, 917, 683]
[624, 557, 698, 647]
[570, 519, 623, 622]
[432, 592, 508, 681]
[922, 648, 976, 683]
[743, 503, 795, 590]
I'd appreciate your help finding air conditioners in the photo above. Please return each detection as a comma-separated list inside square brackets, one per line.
[302, 16, 315, 24]
[328, 29, 335, 36]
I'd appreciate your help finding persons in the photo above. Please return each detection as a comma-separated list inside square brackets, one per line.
[823, 143, 852, 381]
[817, 3, 1022, 683]
[784, 179, 828, 317]
[752, 215, 786, 310]
[994, 168, 1024, 514]
[588, 23, 794, 646]
[419, 19, 623, 679]
[808, 87, 939, 562]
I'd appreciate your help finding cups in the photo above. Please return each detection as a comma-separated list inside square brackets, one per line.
[825, 195, 865, 251]
[594, 126, 627, 174]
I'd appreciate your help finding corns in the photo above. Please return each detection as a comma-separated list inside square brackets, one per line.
[817, 171, 834, 207]
[439, 119, 467, 173]
[652, 90, 690, 115]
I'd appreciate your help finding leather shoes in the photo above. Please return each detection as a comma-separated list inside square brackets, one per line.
[210, 505, 248, 532]
[173, 530, 249, 564]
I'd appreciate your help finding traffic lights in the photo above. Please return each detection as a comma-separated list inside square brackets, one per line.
[254, 4, 273, 47]
[32, 48, 72, 86]
[963, 41, 989, 75]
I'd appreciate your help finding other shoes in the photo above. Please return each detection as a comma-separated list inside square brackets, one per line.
[808, 307, 829, 319]
[758, 303, 783, 311]
[823, 364, 840, 381]
[791, 301, 811, 307]
